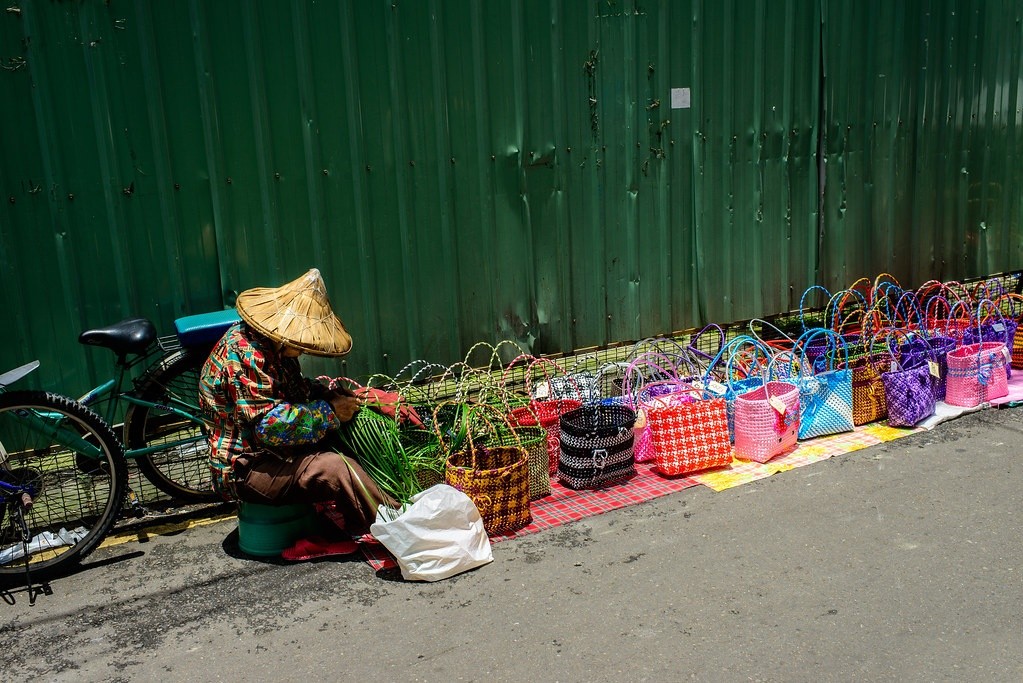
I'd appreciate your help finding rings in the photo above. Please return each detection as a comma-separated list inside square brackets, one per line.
[356, 406, 359, 411]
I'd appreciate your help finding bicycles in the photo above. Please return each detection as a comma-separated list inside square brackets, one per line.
[0, 307, 249, 606]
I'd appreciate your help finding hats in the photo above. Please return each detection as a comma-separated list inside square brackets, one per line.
[236, 268, 352, 357]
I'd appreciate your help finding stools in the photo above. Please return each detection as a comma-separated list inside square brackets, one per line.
[236, 500, 299, 556]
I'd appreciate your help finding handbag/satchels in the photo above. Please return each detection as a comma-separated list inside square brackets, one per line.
[315, 272, 1023, 541]
[370, 484, 493, 583]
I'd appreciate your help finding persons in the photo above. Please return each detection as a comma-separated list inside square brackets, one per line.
[199, 268, 406, 522]
